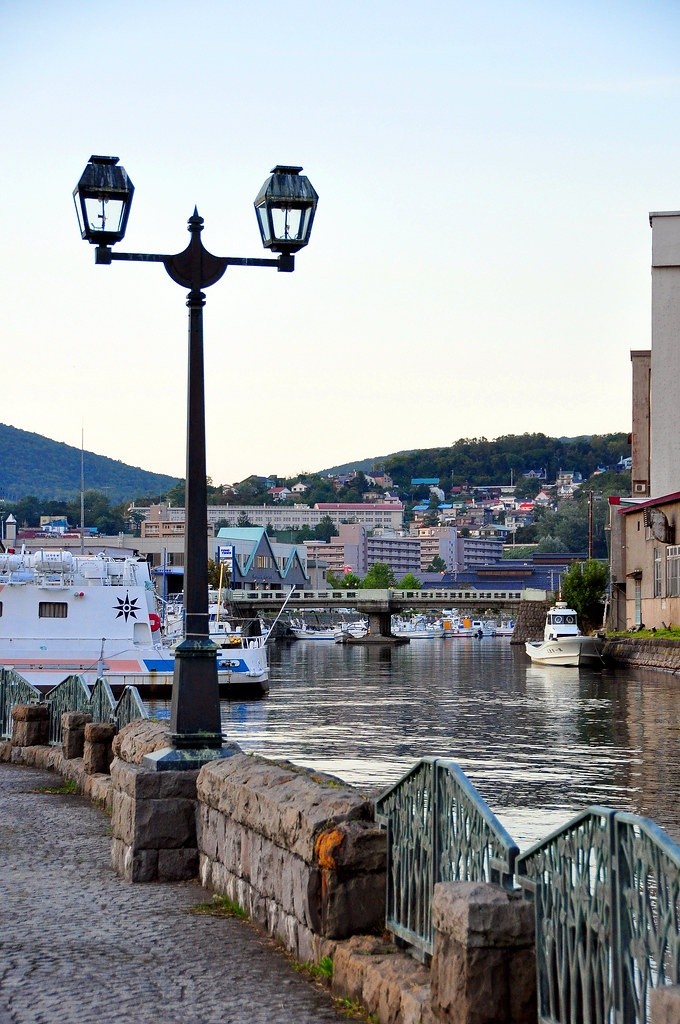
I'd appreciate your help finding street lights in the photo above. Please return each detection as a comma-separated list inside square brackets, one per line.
[70, 154, 319, 769]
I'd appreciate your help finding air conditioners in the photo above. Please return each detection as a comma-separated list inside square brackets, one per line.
[634, 484, 645, 492]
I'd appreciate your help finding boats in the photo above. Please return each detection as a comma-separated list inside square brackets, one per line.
[523, 602, 605, 667]
[0, 551, 273, 696]
[290, 616, 515, 641]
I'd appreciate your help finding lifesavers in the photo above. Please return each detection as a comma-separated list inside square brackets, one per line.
[566, 616, 574, 624]
[149, 614, 161, 631]
[555, 616, 562, 624]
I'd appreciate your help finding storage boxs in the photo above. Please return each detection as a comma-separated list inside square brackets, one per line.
[609, 496, 620, 505]
